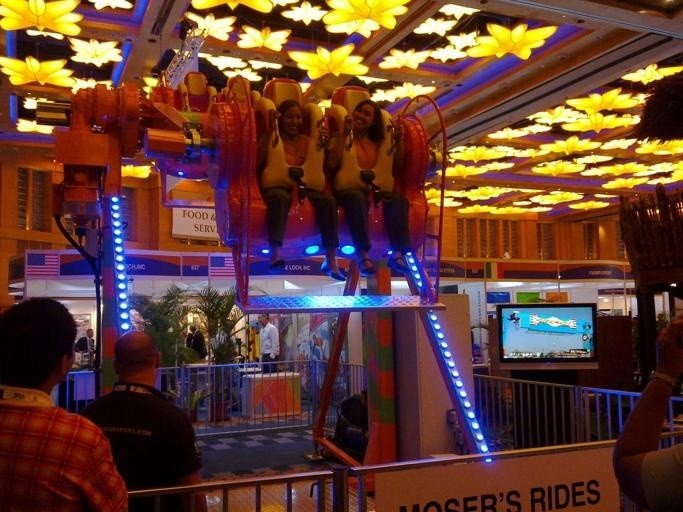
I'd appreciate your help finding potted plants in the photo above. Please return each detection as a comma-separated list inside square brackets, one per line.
[190, 282, 252, 423]
[127, 282, 199, 423]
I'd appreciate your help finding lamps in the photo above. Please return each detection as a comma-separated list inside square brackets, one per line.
[539, 136, 602, 156]
[449, 146, 506, 162]
[435, 164, 490, 179]
[528, 190, 584, 206]
[0, 0, 84, 40]
[525, 106, 584, 122]
[281, 44, 372, 79]
[621, 64, 665, 86]
[378, 47, 433, 70]
[237, 25, 293, 53]
[67, 36, 124, 68]
[578, 161, 650, 178]
[565, 88, 639, 113]
[316, 1, 415, 31]
[561, 114, 635, 134]
[531, 161, 587, 174]
[458, 187, 502, 202]
[465, 21, 560, 62]
[185, 0, 275, 15]
[183, 13, 236, 42]
[0, 55, 79, 88]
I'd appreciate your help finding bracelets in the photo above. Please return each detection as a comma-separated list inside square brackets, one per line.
[650, 369, 678, 388]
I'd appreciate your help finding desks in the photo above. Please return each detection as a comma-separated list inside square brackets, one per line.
[489, 359, 600, 452]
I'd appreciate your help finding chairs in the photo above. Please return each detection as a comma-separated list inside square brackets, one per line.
[178, 71, 217, 123]
[322, 83, 399, 224]
[255, 80, 332, 221]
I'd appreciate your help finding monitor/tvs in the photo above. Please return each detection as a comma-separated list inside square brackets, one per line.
[496, 303, 598, 363]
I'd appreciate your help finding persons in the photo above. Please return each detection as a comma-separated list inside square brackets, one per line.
[327, 99, 411, 283]
[186, 325, 207, 359]
[258, 314, 281, 372]
[74, 329, 95, 352]
[613, 320, 683, 512]
[257, 100, 346, 281]
[1, 298, 129, 511]
[77, 332, 207, 512]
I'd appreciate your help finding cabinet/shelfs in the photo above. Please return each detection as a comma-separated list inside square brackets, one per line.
[242, 372, 302, 420]
